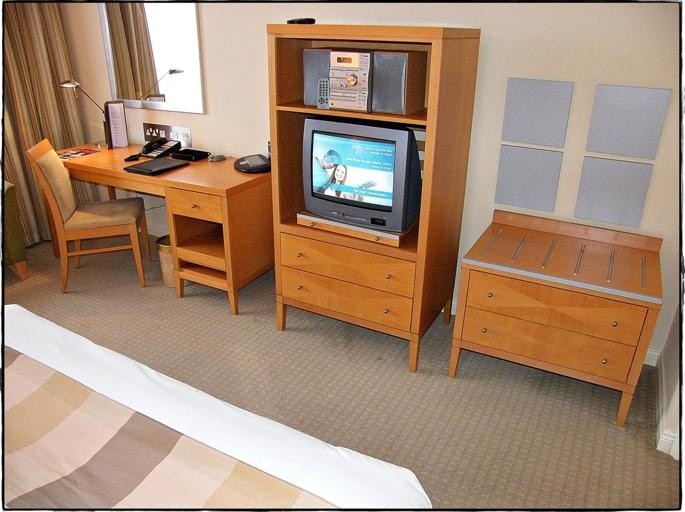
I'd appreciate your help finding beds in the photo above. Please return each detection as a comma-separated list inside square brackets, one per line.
[3, 304, 431, 509]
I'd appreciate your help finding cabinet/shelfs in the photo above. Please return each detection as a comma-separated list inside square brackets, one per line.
[447, 209, 663, 428]
[40, 141, 275, 315]
[267, 24, 481, 373]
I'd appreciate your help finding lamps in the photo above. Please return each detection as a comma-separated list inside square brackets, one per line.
[58, 77, 109, 142]
[138, 68, 184, 100]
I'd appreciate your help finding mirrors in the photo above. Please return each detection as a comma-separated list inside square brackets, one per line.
[97, 2, 206, 115]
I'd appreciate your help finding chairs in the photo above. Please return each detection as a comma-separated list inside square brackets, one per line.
[25, 138, 151, 294]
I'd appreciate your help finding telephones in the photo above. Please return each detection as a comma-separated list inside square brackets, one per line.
[140, 137, 181, 158]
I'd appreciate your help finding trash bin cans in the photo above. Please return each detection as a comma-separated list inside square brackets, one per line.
[156, 234, 191, 287]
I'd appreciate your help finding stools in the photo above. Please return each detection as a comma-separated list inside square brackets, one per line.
[3, 180, 29, 282]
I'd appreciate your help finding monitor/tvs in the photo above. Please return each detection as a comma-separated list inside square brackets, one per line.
[302, 118, 422, 232]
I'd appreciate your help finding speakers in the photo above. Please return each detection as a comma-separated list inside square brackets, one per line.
[303, 48, 331, 106]
[372, 51, 427, 114]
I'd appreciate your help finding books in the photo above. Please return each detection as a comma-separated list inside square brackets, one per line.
[124, 157, 191, 176]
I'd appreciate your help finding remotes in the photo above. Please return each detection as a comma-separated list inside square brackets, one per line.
[317, 77, 329, 109]
[287, 18, 315, 24]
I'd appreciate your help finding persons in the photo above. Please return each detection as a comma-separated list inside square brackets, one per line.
[313, 149, 341, 193]
[317, 162, 365, 202]
